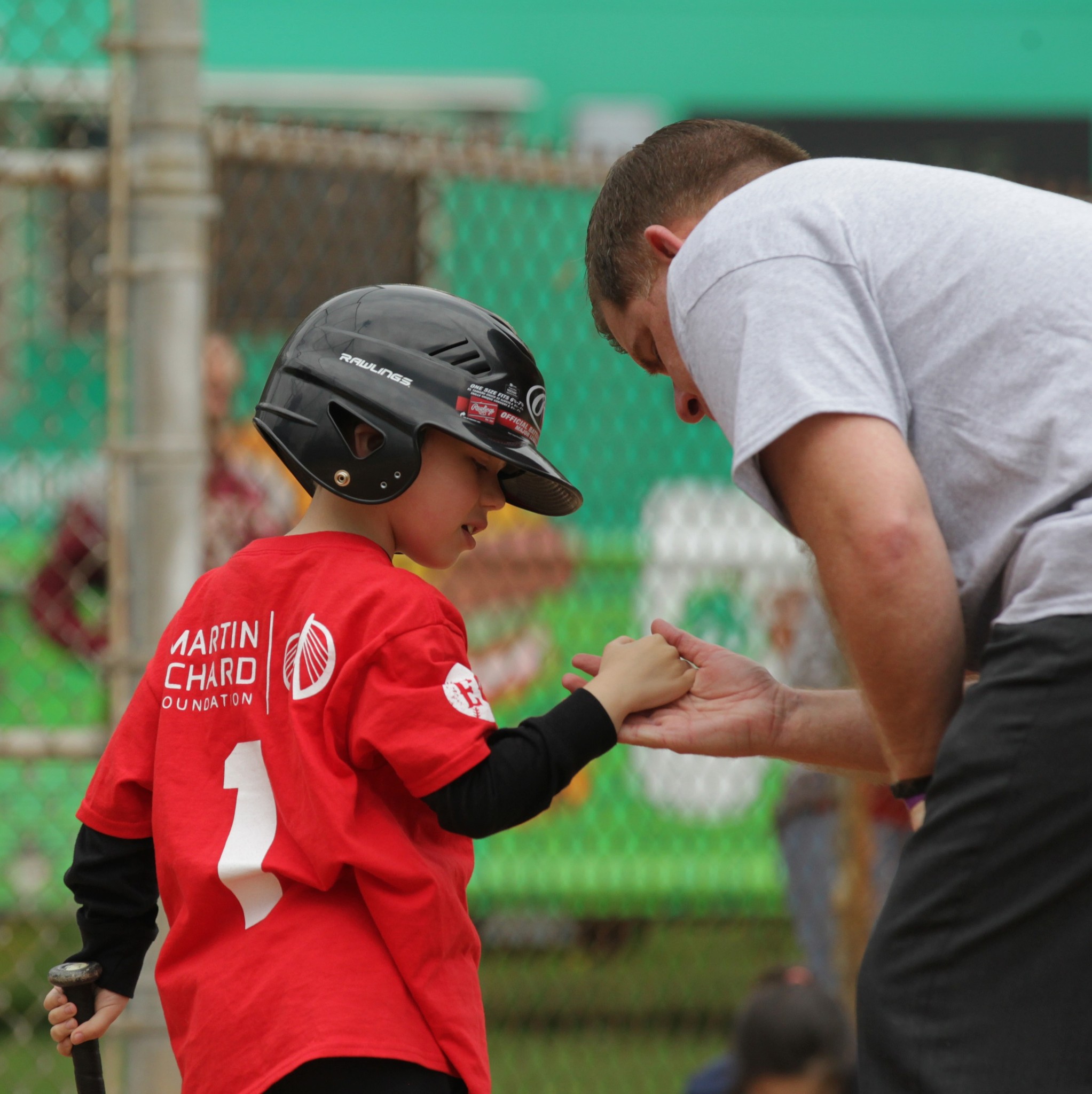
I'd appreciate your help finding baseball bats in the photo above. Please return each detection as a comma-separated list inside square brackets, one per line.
[49, 961, 106, 1094]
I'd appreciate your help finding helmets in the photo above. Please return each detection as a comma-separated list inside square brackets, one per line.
[254, 282, 584, 518]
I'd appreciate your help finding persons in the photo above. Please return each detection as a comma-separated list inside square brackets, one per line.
[562, 121, 1091, 1094]
[43, 286, 695, 1094]
[28, 338, 296, 658]
[688, 967, 852, 1094]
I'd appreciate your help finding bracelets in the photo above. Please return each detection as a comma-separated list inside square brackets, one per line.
[891, 776, 931, 807]
